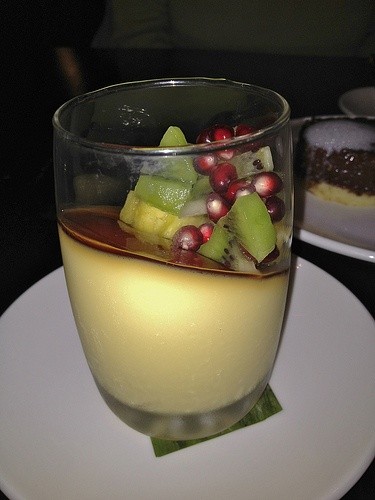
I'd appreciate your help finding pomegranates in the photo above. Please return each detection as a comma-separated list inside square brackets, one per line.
[173, 122, 286, 253]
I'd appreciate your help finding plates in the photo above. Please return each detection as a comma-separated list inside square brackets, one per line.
[1, 255, 375, 500]
[338, 86, 375, 118]
[289, 116, 375, 262]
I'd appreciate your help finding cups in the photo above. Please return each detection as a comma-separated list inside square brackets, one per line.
[52, 78, 295, 442]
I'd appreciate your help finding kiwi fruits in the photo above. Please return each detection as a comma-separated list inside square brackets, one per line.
[115, 125, 278, 274]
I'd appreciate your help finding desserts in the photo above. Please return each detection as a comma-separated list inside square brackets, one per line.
[294, 115, 375, 208]
